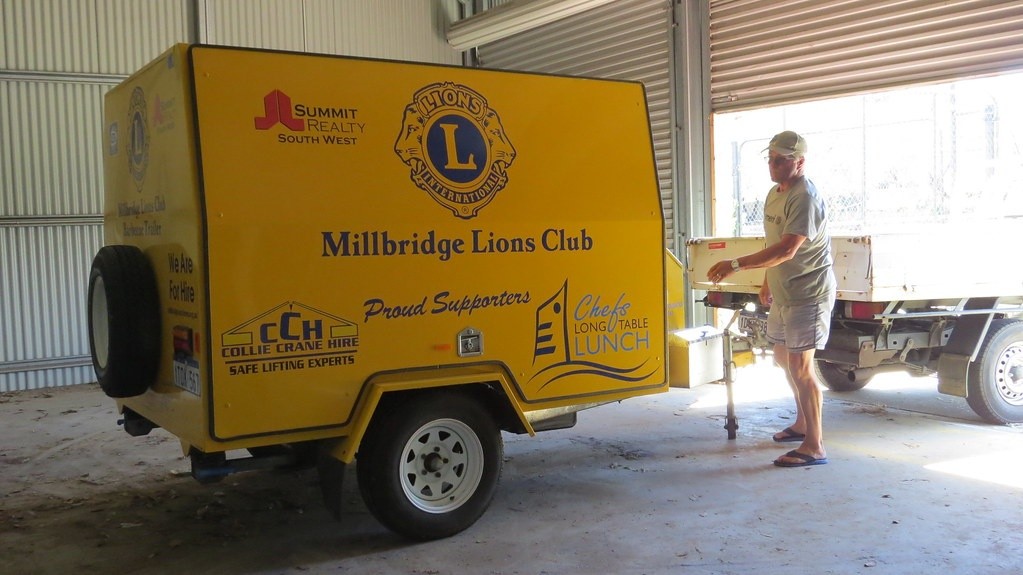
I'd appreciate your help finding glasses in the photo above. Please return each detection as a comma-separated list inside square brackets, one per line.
[765, 155, 790, 165]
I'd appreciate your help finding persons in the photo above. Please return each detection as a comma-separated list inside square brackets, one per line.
[706, 130, 839, 468]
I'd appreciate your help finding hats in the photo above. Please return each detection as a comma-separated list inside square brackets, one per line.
[760, 131, 807, 160]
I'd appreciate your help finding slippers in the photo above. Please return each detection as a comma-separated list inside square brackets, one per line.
[773, 449, 827, 468]
[772, 427, 806, 441]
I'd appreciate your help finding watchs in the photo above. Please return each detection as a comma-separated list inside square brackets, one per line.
[731, 258, 739, 272]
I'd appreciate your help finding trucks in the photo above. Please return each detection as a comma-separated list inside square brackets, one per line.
[684, 218, 1022, 428]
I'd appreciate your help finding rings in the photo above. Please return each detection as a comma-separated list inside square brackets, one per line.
[717, 273, 722, 279]
